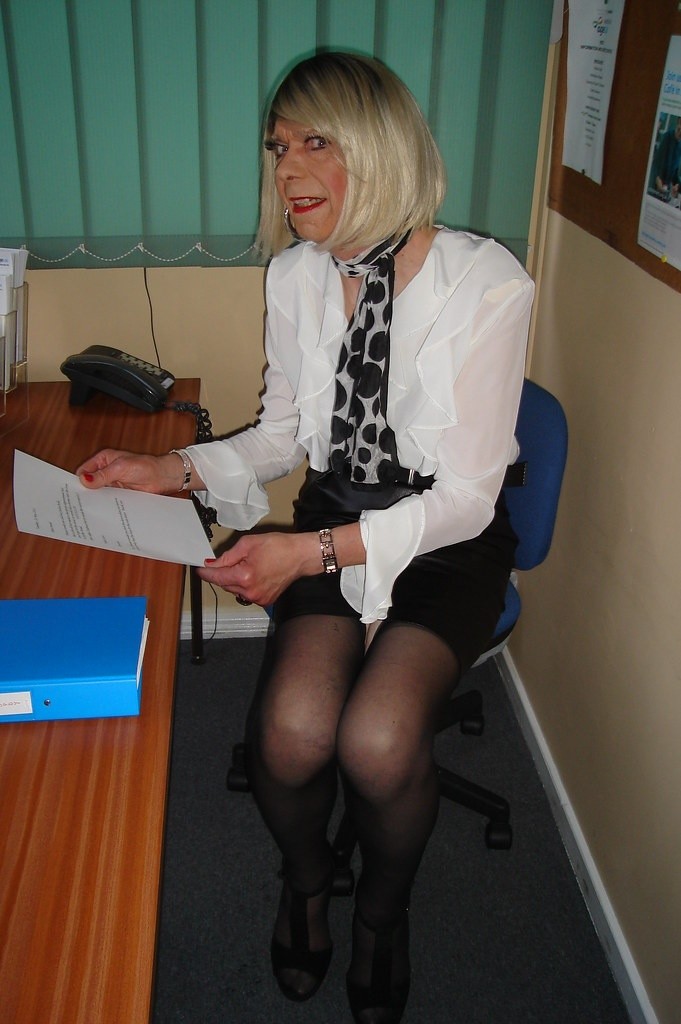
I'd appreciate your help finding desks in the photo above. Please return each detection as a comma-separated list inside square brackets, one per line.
[0, 376, 202, 1024]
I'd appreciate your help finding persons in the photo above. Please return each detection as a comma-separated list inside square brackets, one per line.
[71, 44, 540, 1023]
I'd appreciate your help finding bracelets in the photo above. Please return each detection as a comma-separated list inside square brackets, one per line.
[169, 450, 192, 493]
[318, 529, 338, 575]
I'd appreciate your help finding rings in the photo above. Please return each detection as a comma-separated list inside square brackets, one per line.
[236, 593, 252, 607]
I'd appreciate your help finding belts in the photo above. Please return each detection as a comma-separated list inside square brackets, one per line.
[398, 461, 527, 488]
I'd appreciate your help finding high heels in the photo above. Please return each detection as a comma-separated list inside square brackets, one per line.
[347, 906, 411, 1024]
[271, 841, 336, 1002]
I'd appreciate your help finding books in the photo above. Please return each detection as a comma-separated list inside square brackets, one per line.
[0, 596, 151, 723]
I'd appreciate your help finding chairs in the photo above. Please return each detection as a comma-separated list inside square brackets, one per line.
[228, 377, 568, 899]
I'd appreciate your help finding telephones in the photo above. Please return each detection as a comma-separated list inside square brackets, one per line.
[60, 345, 176, 415]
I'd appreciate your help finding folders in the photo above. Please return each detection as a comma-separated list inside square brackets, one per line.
[0, 597, 150, 723]
